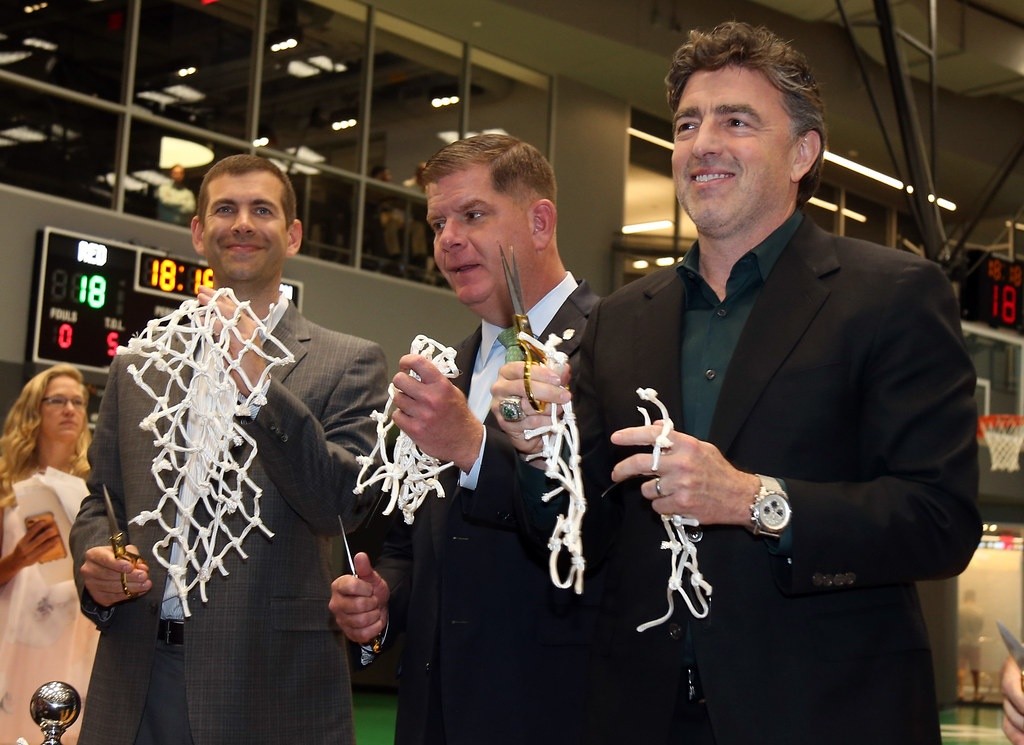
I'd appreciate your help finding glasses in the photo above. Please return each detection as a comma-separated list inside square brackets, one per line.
[41, 395, 88, 410]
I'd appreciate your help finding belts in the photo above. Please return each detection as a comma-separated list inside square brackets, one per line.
[157, 619, 185, 646]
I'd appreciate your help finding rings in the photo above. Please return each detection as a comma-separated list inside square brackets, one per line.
[654, 478, 665, 497]
[499, 395, 527, 423]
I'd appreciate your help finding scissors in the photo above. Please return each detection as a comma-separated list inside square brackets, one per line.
[498, 241, 571, 416]
[332, 512, 390, 658]
[99, 481, 150, 599]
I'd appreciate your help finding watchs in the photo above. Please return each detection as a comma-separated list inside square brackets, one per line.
[743, 473, 793, 539]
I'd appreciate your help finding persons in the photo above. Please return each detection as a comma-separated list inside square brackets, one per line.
[0, 364, 95, 745]
[490, 18, 984, 745]
[1000, 642, 1024, 745]
[69, 132, 601, 745]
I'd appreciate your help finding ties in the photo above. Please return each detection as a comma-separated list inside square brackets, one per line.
[496, 326, 526, 365]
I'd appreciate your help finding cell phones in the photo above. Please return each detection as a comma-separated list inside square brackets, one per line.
[25, 511, 67, 564]
